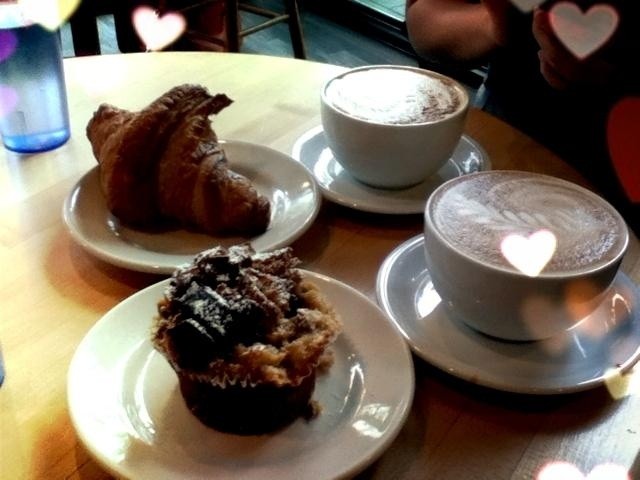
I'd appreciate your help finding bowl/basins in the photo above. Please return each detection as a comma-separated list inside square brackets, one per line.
[322, 65, 469, 188]
[427, 171, 630, 344]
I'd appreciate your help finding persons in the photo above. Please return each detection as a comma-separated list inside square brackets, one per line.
[405, 0, 640, 243]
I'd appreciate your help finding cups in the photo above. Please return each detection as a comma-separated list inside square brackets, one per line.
[1, 4, 71, 154]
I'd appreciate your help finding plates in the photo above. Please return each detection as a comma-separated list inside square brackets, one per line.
[376, 231, 640, 395]
[68, 278, 412, 480]
[291, 126, 491, 215]
[60, 140, 326, 278]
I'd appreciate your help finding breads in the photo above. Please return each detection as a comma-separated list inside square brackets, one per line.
[149, 242, 342, 437]
[86, 81, 272, 237]
